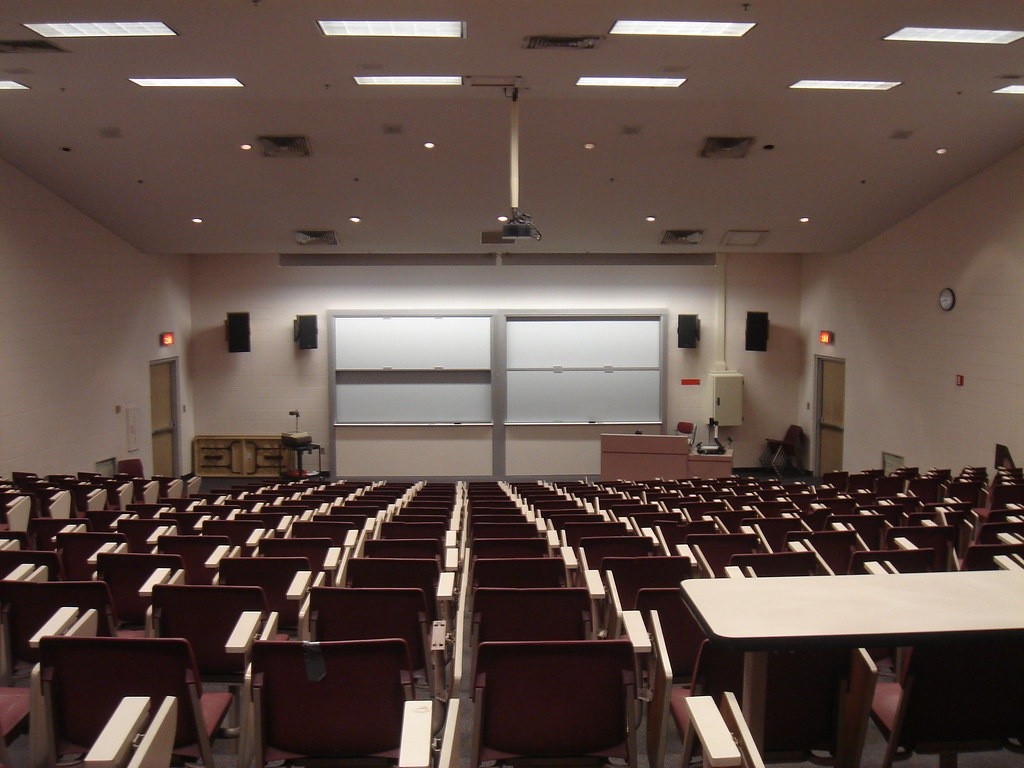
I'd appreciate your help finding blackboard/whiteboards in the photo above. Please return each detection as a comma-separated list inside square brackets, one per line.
[325, 309, 496, 427]
[503, 309, 669, 427]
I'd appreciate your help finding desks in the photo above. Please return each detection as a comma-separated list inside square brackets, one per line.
[680, 570, 1024, 768]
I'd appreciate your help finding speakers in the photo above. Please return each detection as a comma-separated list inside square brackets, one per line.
[678, 314, 698, 349]
[227, 311, 251, 352]
[298, 315, 318, 349]
[746, 311, 769, 351]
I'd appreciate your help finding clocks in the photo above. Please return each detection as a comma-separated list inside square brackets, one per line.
[939, 288, 956, 311]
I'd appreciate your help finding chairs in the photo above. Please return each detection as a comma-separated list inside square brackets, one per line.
[0, 467, 1024, 768]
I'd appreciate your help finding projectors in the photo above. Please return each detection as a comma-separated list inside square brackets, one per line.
[503, 224, 533, 239]
